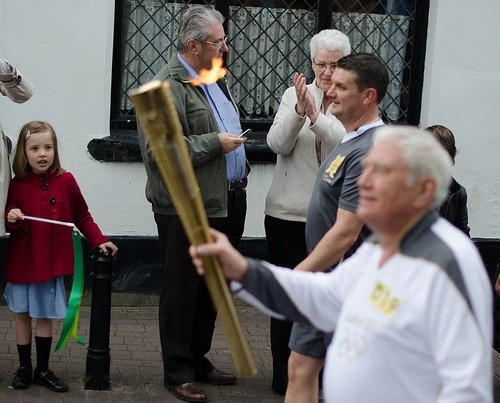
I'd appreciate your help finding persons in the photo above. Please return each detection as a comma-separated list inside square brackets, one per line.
[0, 59, 33, 236]
[138, 6, 253, 403]
[422, 125, 471, 239]
[263, 28, 353, 393]
[3, 121, 118, 393]
[283, 52, 388, 403]
[188, 123, 495, 403]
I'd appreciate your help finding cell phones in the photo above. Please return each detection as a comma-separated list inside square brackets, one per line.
[238, 129, 251, 138]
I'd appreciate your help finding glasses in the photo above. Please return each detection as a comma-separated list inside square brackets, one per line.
[196, 35, 229, 47]
[314, 61, 341, 72]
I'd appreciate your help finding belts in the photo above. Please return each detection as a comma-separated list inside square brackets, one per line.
[227, 178, 249, 190]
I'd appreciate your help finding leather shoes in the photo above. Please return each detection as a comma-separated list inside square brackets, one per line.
[11, 366, 33, 387]
[164, 380, 206, 401]
[195, 368, 236, 385]
[33, 370, 69, 392]
